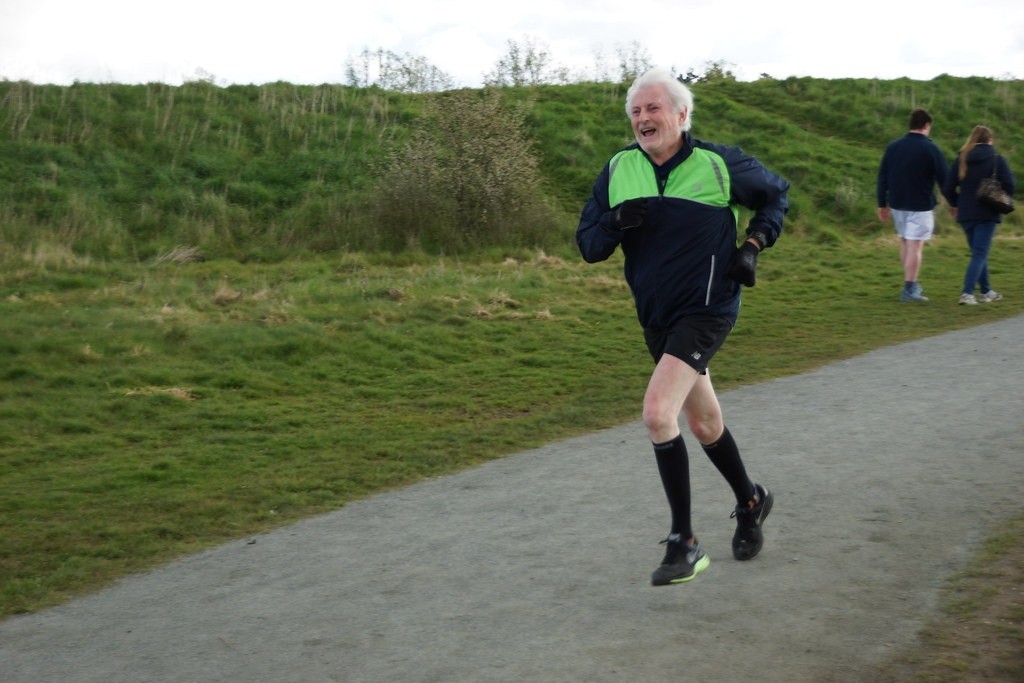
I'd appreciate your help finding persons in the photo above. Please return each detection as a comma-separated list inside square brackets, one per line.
[577, 68, 790, 585]
[878, 110, 959, 302]
[949, 125, 1014, 305]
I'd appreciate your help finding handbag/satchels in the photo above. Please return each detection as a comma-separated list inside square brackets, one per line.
[975, 180, 1015, 215]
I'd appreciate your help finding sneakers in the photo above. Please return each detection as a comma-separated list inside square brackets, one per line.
[907, 282, 922, 294]
[957, 293, 979, 304]
[652, 532, 710, 586]
[729, 484, 772, 559]
[978, 290, 1003, 302]
[901, 291, 928, 303]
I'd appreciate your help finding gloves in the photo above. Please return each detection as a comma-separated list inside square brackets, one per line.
[610, 199, 653, 231]
[724, 240, 760, 287]
